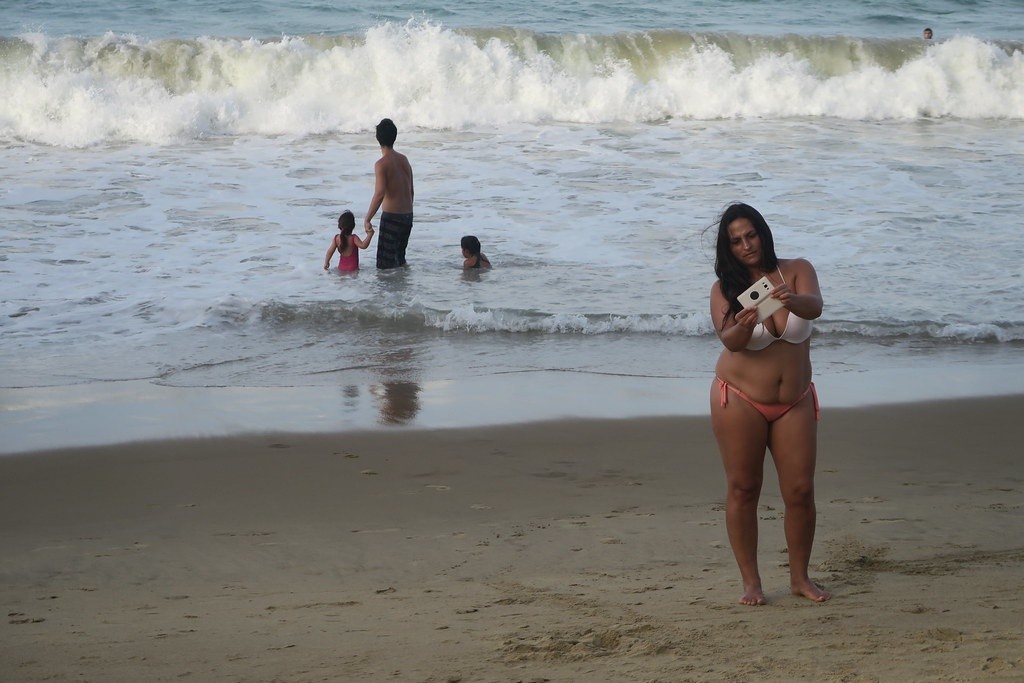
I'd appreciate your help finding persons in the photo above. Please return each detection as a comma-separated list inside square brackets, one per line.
[364, 118, 415, 270]
[323, 212, 375, 272]
[460, 235, 492, 269]
[708, 203, 833, 607]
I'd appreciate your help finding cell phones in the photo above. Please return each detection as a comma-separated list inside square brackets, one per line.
[737, 276, 774, 310]
